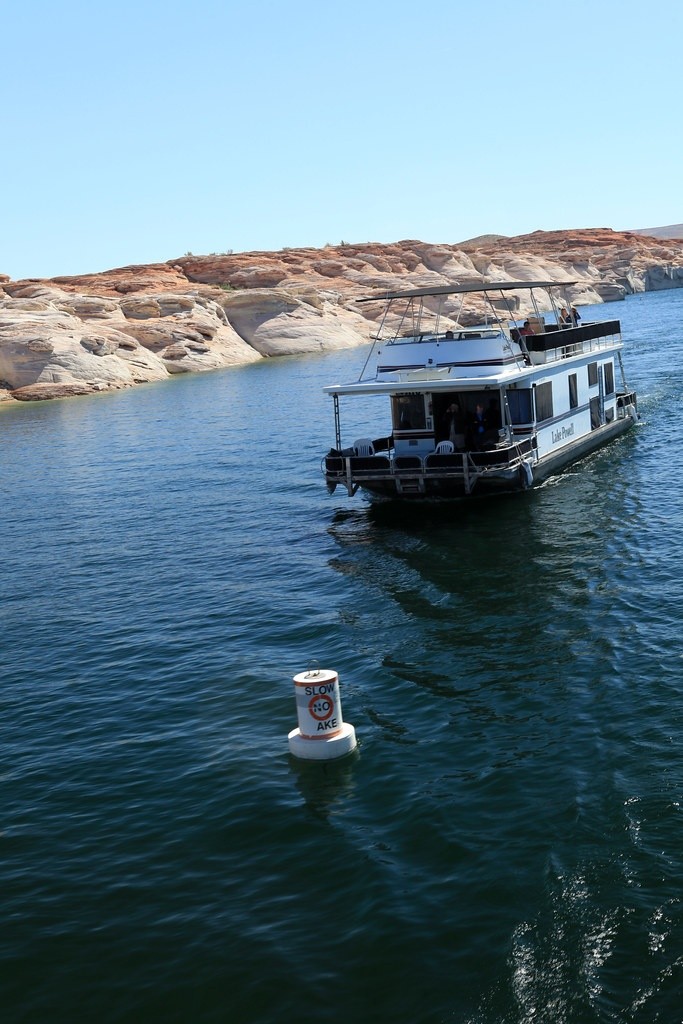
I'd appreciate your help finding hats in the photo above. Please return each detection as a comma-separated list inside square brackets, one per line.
[449, 404, 459, 410]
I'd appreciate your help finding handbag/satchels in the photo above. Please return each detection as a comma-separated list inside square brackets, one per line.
[465, 424, 482, 451]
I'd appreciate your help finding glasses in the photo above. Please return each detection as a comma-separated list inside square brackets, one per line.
[573, 311, 575, 313]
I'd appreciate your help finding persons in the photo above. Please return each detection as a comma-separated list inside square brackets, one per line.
[559, 307, 581, 324]
[442, 404, 465, 448]
[485, 398, 499, 421]
[471, 402, 488, 451]
[520, 321, 533, 335]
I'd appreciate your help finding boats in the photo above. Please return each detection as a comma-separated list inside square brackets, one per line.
[323, 285, 639, 489]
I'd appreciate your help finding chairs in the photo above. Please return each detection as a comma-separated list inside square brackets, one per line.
[429, 441, 454, 454]
[354, 439, 375, 457]
